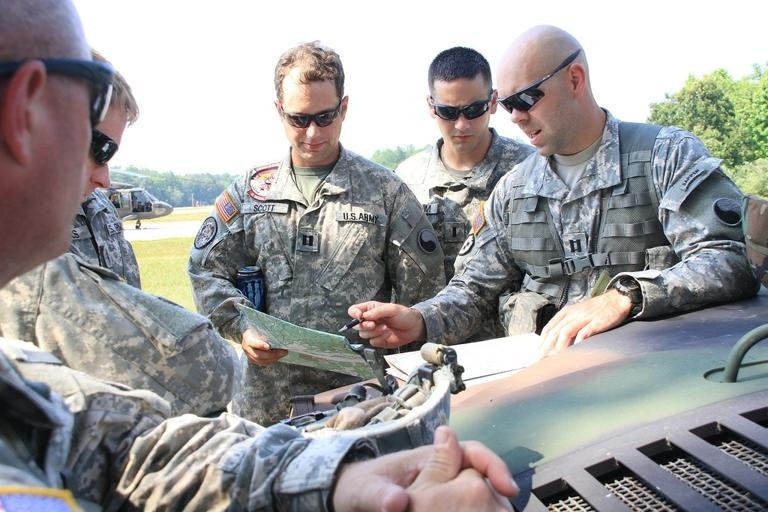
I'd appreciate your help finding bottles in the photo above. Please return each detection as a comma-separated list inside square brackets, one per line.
[236, 266, 264, 312]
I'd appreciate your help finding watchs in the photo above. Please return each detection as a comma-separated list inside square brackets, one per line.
[611, 277, 642, 321]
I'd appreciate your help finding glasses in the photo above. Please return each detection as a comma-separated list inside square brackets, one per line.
[2, 54, 115, 123]
[498, 49, 582, 114]
[91, 128, 119, 162]
[279, 100, 345, 127]
[430, 94, 493, 120]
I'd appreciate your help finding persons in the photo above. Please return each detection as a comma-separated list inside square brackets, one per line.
[190, 43, 444, 430]
[1, 0, 519, 512]
[396, 49, 541, 343]
[0, 49, 241, 416]
[348, 26, 759, 352]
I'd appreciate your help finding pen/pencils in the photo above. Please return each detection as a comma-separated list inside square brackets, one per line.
[338, 317, 361, 334]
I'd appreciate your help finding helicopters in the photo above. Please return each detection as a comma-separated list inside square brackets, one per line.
[110, 181, 172, 228]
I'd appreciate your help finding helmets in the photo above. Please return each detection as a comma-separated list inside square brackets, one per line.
[742, 195, 768, 288]
[278, 342, 466, 463]
